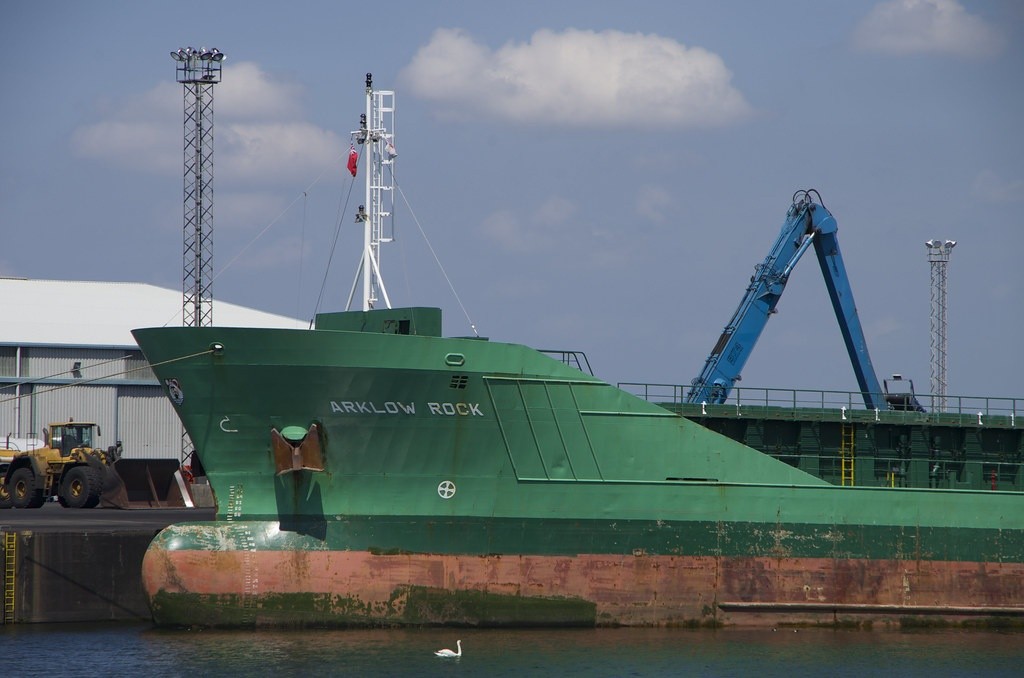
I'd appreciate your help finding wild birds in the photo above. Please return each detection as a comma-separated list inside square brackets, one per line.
[433, 639, 463, 658]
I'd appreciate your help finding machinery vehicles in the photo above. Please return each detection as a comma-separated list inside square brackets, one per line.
[2, 416, 199, 510]
[0, 432, 25, 509]
[682, 186, 896, 411]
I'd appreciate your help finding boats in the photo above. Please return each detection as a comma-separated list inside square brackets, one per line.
[130, 72, 1024, 633]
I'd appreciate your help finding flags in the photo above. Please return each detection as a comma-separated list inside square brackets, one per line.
[346, 144, 358, 177]
[384, 142, 397, 158]
